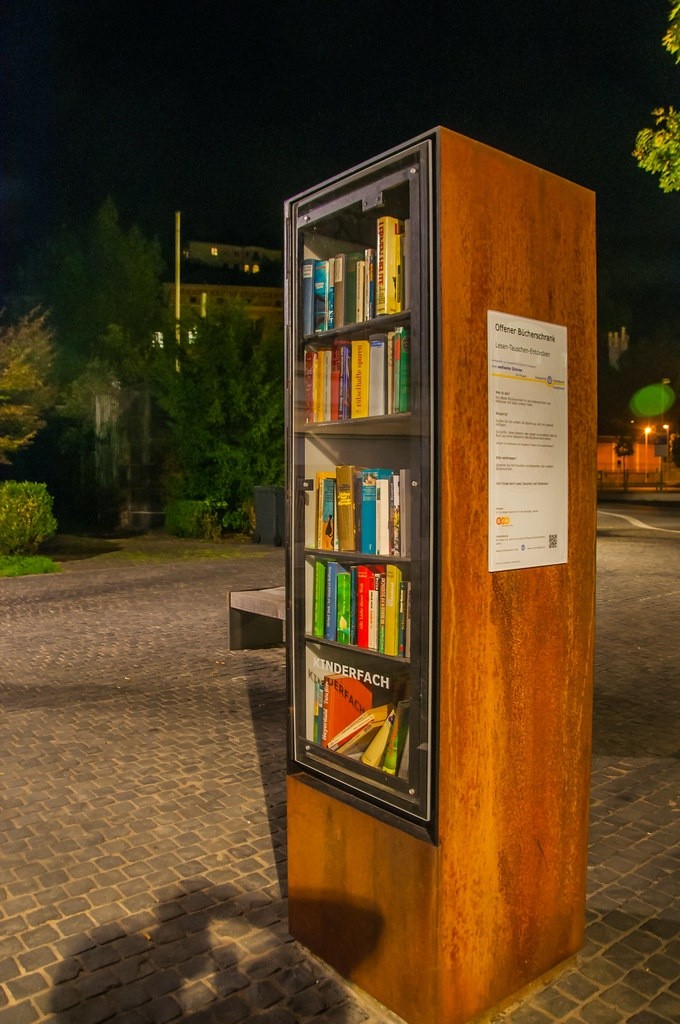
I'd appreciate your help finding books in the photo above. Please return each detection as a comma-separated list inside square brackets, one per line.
[312, 559, 411, 661]
[303, 328, 410, 425]
[315, 466, 406, 558]
[299, 217, 410, 338]
[313, 667, 408, 788]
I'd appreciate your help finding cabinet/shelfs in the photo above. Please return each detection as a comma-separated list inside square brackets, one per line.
[290, 142, 434, 823]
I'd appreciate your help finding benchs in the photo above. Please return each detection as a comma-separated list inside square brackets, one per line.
[228, 584, 285, 642]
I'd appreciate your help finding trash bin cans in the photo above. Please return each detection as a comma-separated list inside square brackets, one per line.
[249, 485, 285, 547]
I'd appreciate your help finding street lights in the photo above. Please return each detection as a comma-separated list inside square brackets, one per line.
[660, 378, 670, 491]
[621, 420, 635, 490]
[644, 426, 650, 482]
[663, 424, 671, 490]
